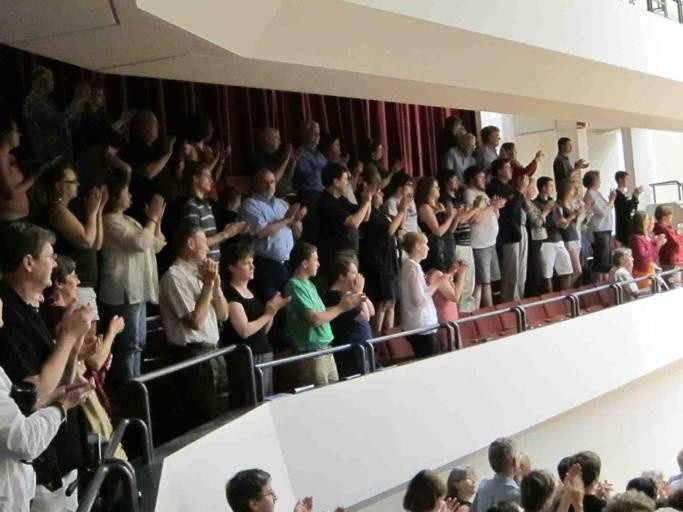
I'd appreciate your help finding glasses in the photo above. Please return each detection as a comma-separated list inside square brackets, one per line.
[58, 178, 79, 186]
[256, 488, 276, 502]
[63, 273, 78, 282]
[32, 253, 59, 263]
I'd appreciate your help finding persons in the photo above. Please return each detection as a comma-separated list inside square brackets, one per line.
[1, 66, 681, 509]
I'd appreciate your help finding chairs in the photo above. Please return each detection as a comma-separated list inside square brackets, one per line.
[368, 280, 629, 369]
